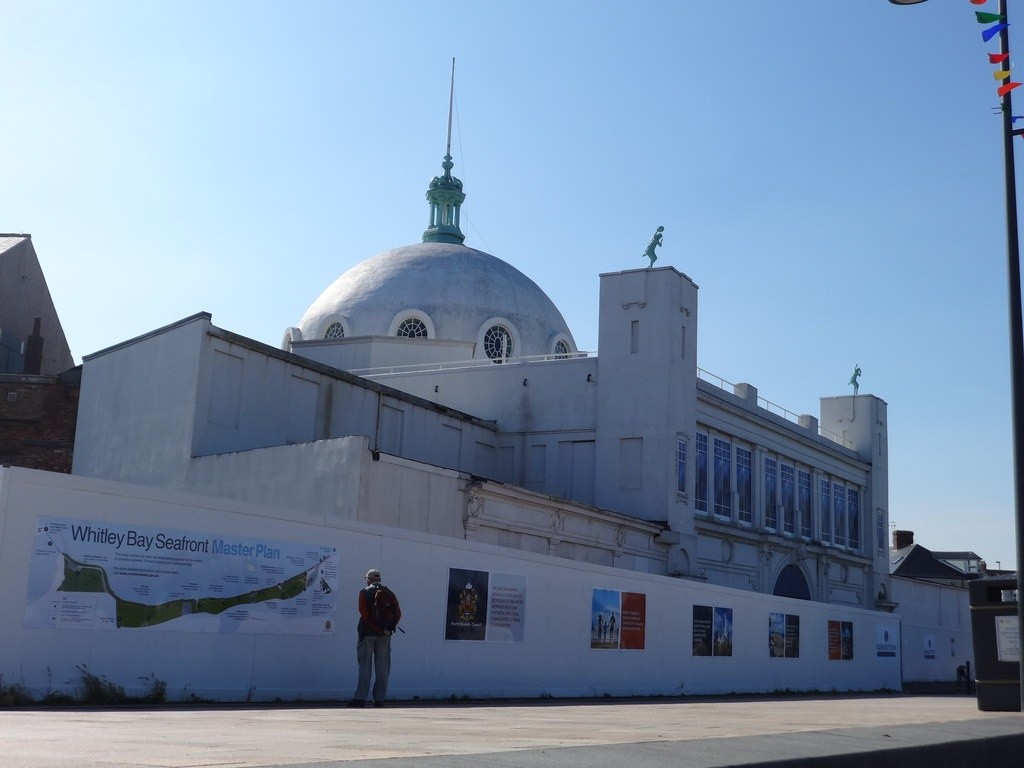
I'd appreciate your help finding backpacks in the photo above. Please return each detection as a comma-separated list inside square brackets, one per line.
[371, 586, 397, 633]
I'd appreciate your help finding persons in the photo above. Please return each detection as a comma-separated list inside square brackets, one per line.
[597, 611, 616, 645]
[847, 363, 862, 395]
[347, 568, 402, 707]
[642, 228, 665, 268]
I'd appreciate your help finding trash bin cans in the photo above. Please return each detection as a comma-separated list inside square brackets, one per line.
[967, 576, 1021, 712]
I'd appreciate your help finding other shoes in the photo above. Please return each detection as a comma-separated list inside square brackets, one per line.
[375, 700, 385, 707]
[345, 699, 364, 708]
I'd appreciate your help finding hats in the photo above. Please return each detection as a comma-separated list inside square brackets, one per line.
[362, 569, 381, 580]
